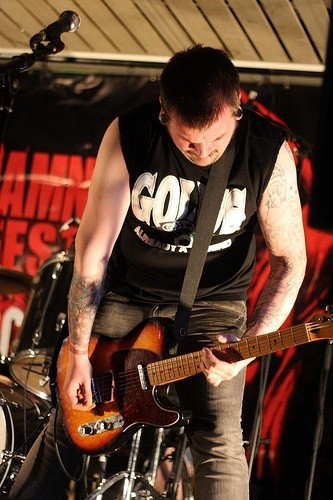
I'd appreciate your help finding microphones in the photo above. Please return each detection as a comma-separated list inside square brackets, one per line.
[30, 11, 81, 50]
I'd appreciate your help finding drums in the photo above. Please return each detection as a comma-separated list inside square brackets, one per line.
[0, 379, 51, 496]
[8, 251, 76, 402]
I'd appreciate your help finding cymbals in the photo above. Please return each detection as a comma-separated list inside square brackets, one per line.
[0, 265, 32, 294]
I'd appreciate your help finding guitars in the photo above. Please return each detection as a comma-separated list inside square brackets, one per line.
[52, 315, 333, 457]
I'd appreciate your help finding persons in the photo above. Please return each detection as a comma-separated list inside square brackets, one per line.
[62, 41, 308, 499]
